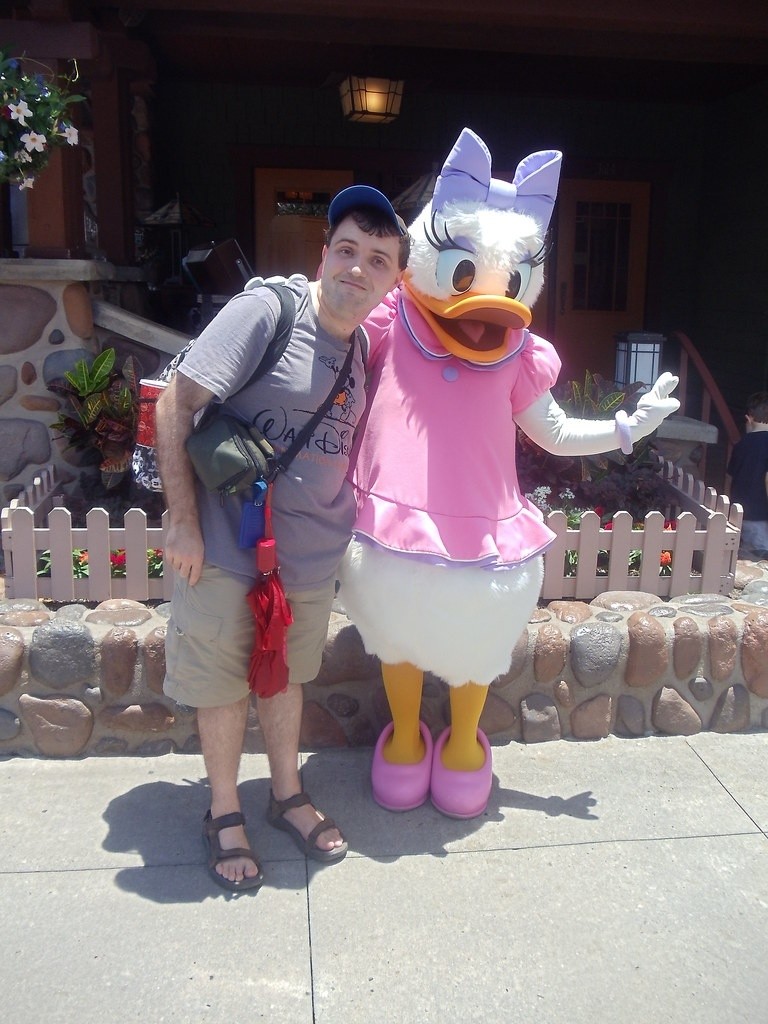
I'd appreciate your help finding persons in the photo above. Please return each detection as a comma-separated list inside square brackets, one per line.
[153, 186, 410, 890]
[723, 390, 768, 557]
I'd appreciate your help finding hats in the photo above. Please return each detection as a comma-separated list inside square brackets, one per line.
[329, 184, 405, 239]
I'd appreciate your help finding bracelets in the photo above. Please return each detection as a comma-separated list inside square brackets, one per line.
[614, 411, 633, 455]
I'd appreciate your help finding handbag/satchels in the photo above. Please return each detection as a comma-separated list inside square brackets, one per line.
[132, 284, 297, 494]
[185, 330, 356, 505]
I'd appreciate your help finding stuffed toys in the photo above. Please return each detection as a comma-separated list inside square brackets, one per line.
[244, 128, 682, 820]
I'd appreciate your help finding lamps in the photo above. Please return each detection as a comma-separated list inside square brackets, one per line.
[334, 53, 409, 125]
[613, 331, 669, 399]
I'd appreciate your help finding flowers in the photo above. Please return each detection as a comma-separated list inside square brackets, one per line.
[0, 43, 86, 191]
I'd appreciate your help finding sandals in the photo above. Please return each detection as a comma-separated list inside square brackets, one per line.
[265, 788, 348, 862]
[201, 809, 265, 889]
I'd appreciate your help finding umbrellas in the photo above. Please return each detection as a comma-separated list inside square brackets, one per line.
[391, 160, 440, 211]
